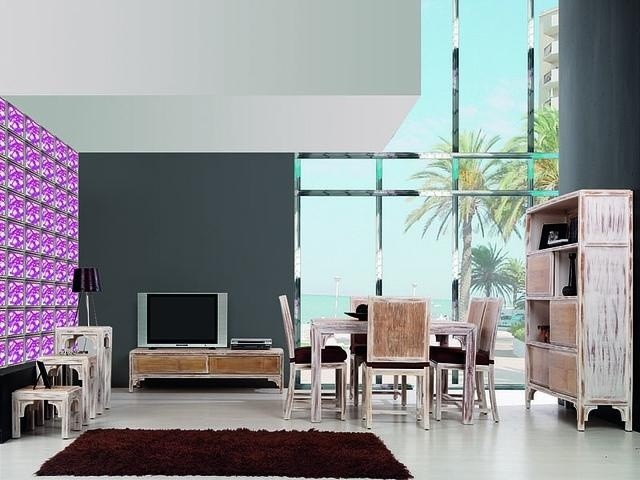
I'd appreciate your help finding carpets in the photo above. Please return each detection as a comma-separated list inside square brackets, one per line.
[30, 427, 412, 479]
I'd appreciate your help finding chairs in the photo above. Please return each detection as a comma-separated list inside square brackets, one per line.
[277, 293, 504, 428]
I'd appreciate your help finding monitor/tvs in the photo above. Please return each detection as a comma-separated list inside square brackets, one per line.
[136, 291, 229, 350]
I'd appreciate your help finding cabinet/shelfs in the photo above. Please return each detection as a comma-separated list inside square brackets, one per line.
[523, 188, 635, 433]
[127, 346, 286, 394]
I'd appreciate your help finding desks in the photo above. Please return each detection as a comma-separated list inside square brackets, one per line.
[35, 326, 112, 423]
[10, 385, 84, 441]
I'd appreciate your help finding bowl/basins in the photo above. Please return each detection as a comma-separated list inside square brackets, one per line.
[345, 312, 367, 321]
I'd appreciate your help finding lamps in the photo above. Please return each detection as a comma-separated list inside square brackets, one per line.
[72, 266, 102, 327]
